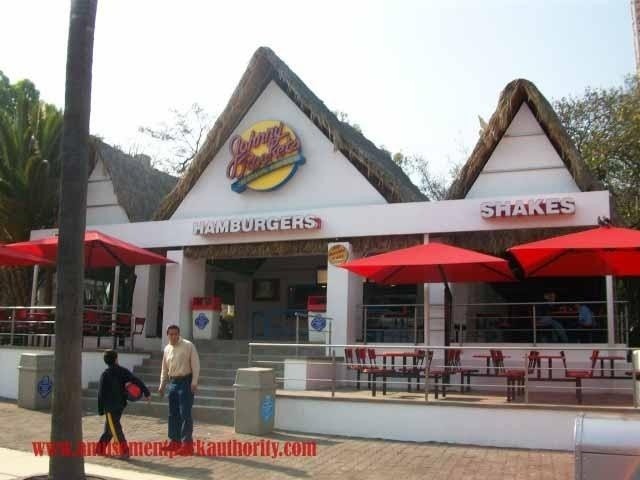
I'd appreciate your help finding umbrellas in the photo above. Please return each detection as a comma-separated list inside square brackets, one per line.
[335, 241, 517, 382]
[505, 227, 640, 278]
[0, 230, 179, 312]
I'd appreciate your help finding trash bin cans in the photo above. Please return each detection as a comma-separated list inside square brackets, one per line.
[307, 293, 326, 343]
[17, 353, 53, 410]
[191, 297, 222, 340]
[233, 368, 275, 435]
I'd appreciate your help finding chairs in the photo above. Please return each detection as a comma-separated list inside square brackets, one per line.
[343, 347, 640, 401]
[0, 305, 146, 351]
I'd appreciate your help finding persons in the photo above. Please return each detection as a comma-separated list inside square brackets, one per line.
[534, 288, 602, 343]
[376, 289, 397, 342]
[158, 326, 200, 457]
[97, 350, 151, 460]
